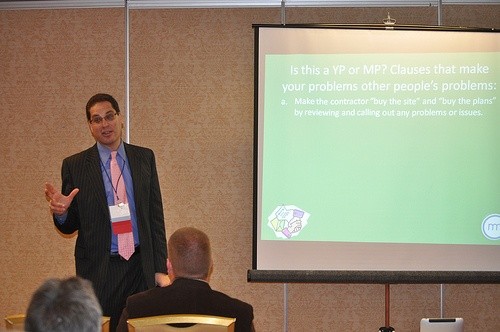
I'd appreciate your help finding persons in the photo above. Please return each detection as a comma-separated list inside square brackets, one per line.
[115, 225, 257, 332]
[25, 275, 100, 332]
[44, 93, 169, 332]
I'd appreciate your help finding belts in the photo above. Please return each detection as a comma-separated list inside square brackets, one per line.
[110, 249, 139, 261]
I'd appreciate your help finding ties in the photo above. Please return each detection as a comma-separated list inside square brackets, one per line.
[110, 151, 135, 260]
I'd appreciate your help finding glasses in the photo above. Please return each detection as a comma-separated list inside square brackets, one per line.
[89, 113, 117, 124]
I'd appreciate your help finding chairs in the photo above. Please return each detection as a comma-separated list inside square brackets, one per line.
[127, 314, 236, 332]
[3, 314, 111, 332]
